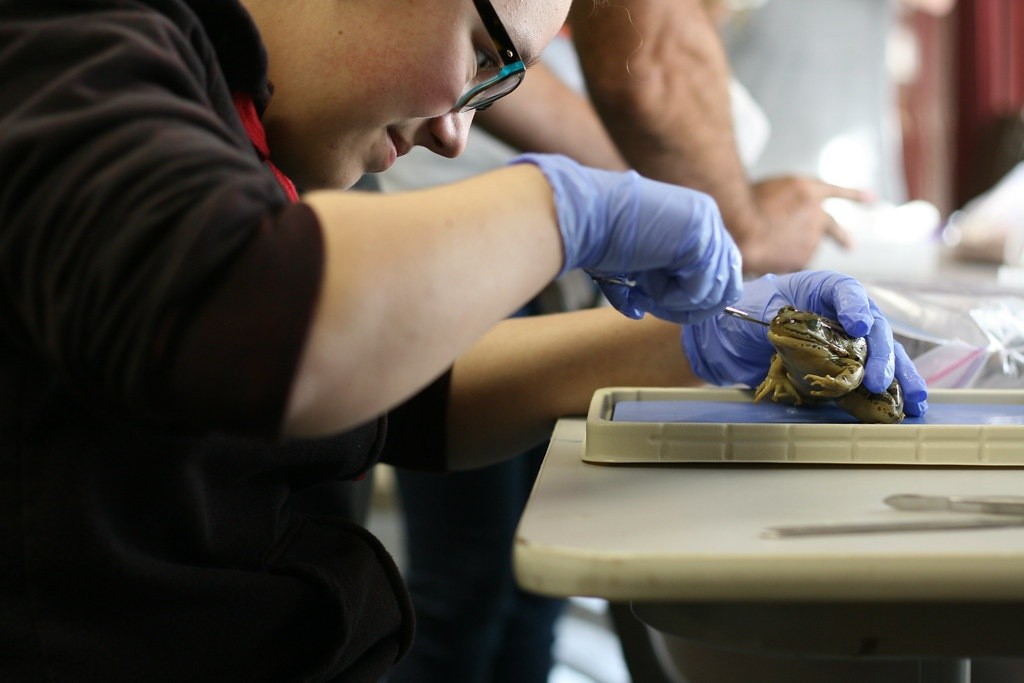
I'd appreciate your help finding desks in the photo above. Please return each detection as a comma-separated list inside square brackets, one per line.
[513, 418, 1024, 683]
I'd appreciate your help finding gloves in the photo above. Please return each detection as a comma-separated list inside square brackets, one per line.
[510, 153, 742, 325]
[681, 269, 930, 422]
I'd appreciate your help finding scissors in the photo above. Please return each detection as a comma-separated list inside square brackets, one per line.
[591, 274, 769, 327]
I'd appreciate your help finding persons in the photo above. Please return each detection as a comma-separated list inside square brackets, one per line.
[476, 1, 883, 285]
[0, 1, 934, 682]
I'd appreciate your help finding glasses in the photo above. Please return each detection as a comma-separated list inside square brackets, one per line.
[456, 1, 527, 113]
